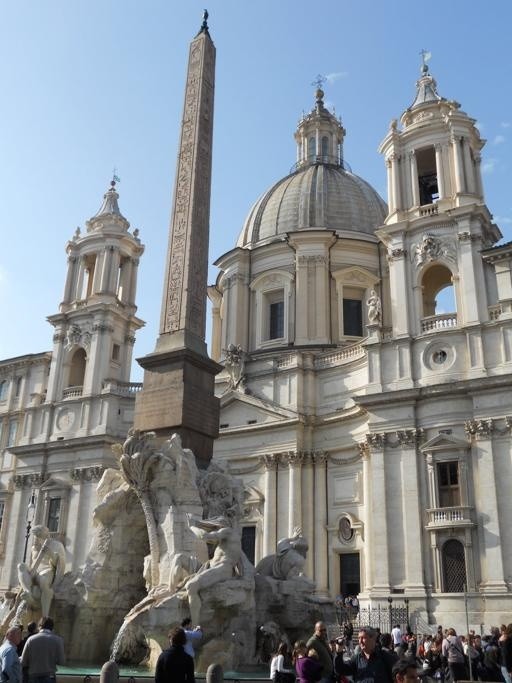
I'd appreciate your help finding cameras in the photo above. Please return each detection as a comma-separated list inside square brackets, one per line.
[336, 638, 344, 645]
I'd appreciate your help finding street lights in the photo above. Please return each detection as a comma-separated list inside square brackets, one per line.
[22, 488, 38, 562]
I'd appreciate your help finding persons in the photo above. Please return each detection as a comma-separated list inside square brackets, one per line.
[256, 526, 309, 579]
[155, 626, 195, 682]
[269, 593, 512, 682]
[23, 616, 67, 683]
[0, 626, 24, 682]
[14, 624, 24, 657]
[17, 524, 66, 626]
[151, 507, 241, 631]
[24, 621, 38, 648]
[180, 617, 202, 656]
